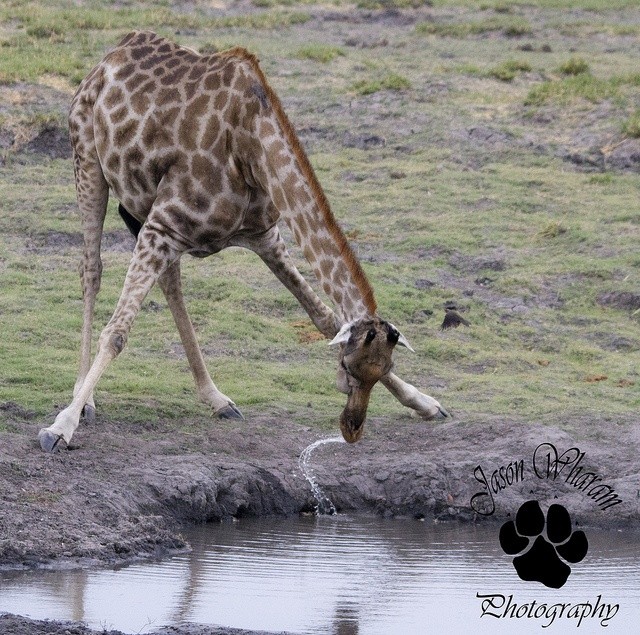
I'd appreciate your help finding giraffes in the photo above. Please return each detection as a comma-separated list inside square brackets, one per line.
[36, 29, 454, 457]
